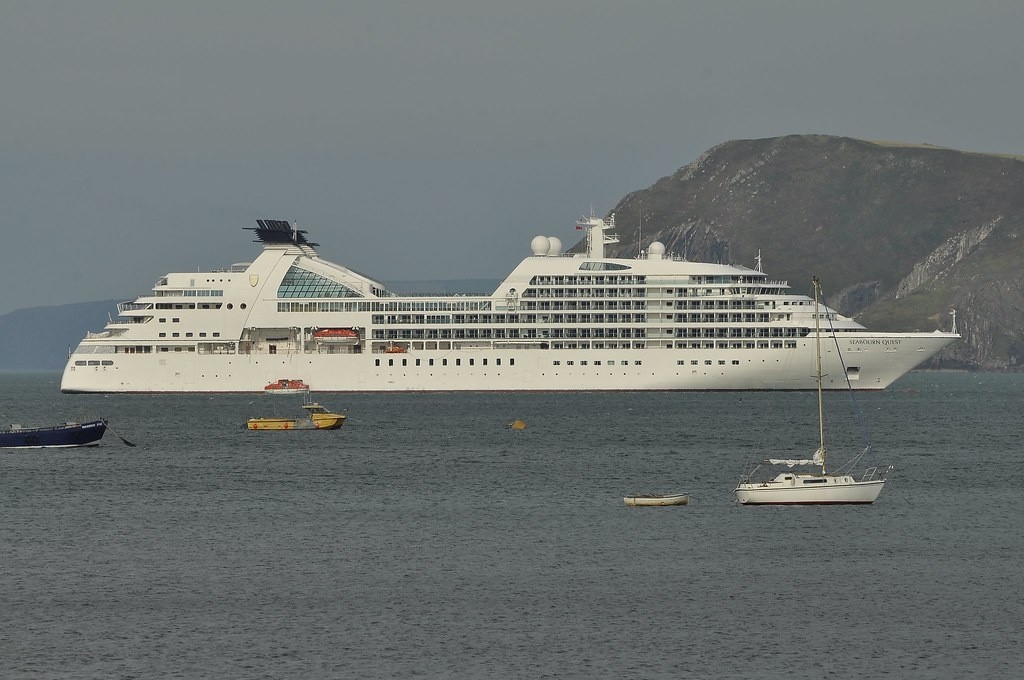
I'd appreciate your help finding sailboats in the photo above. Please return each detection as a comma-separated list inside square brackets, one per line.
[735, 275, 897, 508]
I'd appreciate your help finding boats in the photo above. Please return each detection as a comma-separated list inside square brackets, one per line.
[623, 491, 690, 506]
[247, 402, 348, 430]
[1, 415, 110, 452]
[312, 329, 360, 346]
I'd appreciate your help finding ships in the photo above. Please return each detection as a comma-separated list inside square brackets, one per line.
[60, 216, 961, 391]
